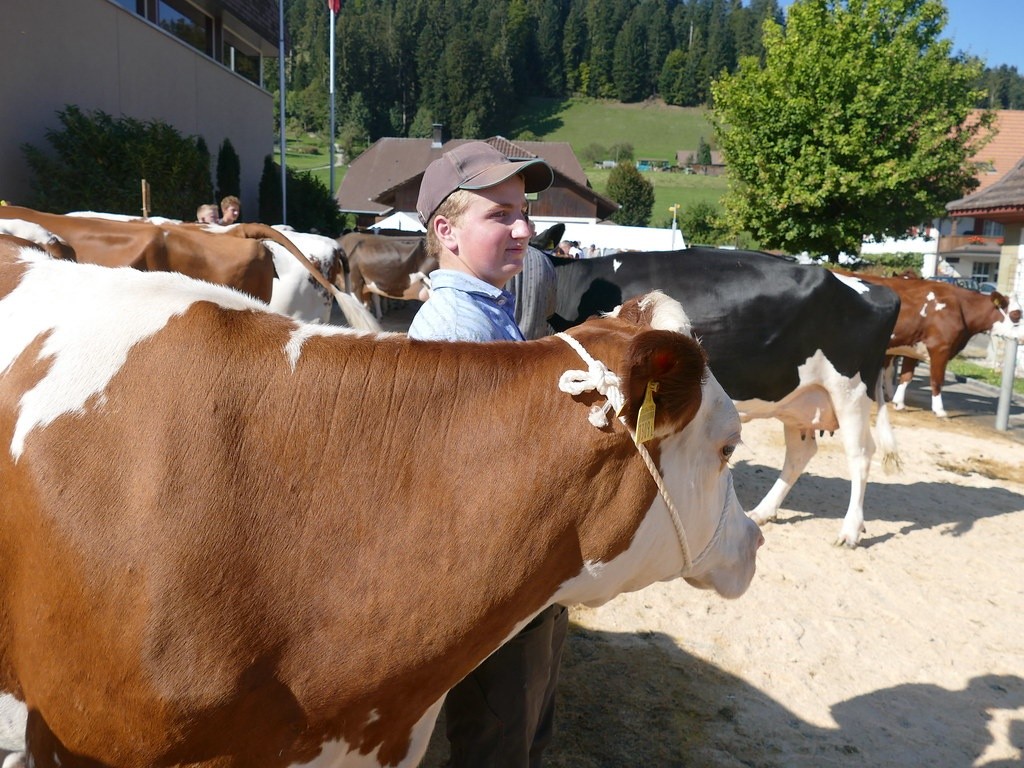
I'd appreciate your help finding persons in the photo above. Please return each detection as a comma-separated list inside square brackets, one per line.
[218, 195, 241, 226]
[197, 204, 219, 225]
[528, 234, 600, 260]
[402, 141, 568, 768]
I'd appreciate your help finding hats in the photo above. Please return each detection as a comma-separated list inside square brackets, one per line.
[416, 141, 554, 229]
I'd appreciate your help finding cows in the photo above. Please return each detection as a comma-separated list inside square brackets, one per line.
[334, 232, 440, 311]
[64, 209, 360, 325]
[1, 201, 384, 335]
[524, 223, 903, 549]
[1, 237, 767, 768]
[826, 268, 1024, 418]
[891, 270, 925, 280]
